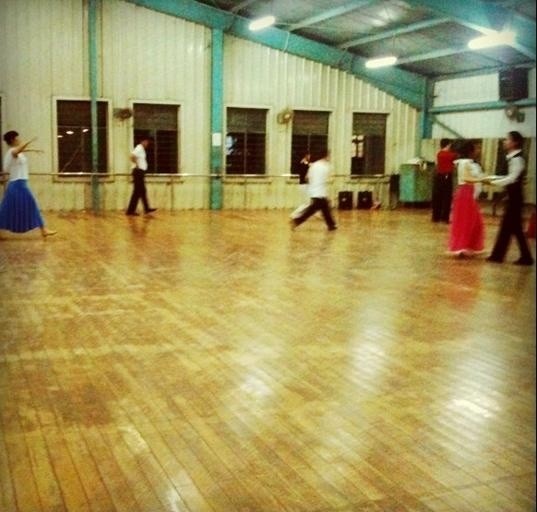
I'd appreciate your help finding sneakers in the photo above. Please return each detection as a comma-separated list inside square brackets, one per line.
[41, 229, 55, 237]
[126, 208, 157, 217]
[289, 216, 300, 229]
[486, 253, 532, 267]
[326, 226, 336, 231]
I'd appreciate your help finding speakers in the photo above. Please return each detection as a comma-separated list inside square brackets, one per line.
[498, 69, 529, 102]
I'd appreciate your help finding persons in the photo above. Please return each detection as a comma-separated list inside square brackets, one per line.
[126, 137, 156, 217]
[289, 150, 324, 221]
[445, 141, 498, 258]
[290, 149, 339, 232]
[0, 130, 58, 237]
[430, 138, 462, 223]
[481, 132, 534, 266]
